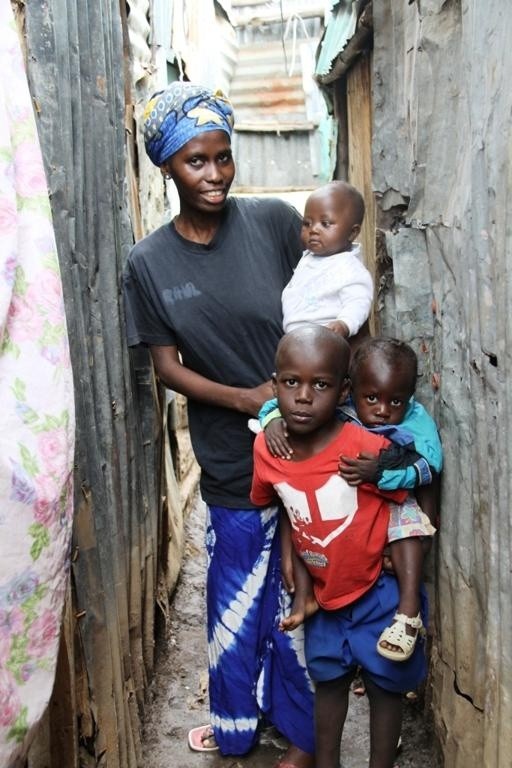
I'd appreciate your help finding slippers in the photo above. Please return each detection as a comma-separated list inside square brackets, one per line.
[186, 723, 224, 752]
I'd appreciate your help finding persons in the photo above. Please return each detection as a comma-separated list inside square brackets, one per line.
[243, 180, 374, 435]
[279, 333, 442, 662]
[246, 324, 425, 766]
[125, 83, 305, 755]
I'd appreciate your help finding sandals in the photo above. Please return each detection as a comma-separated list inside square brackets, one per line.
[376, 606, 425, 663]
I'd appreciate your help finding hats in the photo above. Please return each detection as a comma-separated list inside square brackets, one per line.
[144, 80, 236, 168]
